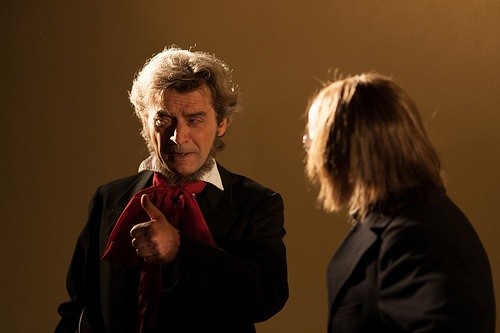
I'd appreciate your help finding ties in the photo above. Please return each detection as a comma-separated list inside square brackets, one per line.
[99, 171, 218, 260]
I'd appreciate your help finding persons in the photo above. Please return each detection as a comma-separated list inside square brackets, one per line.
[303, 74, 496, 333]
[53, 49, 290, 333]
